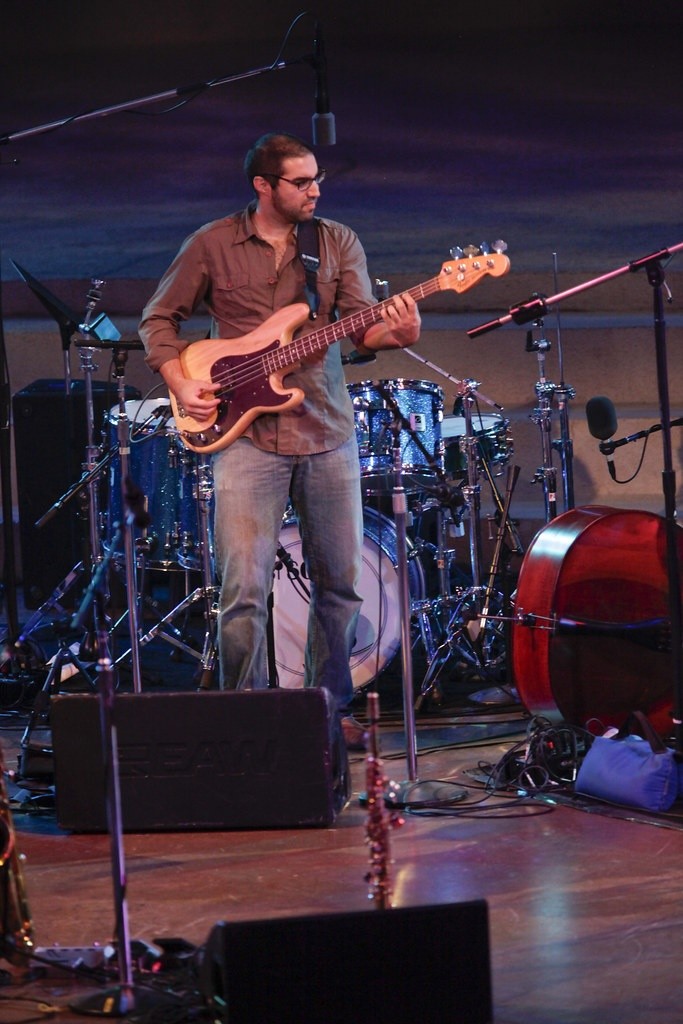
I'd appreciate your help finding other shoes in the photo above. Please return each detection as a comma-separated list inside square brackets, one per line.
[341, 716, 376, 752]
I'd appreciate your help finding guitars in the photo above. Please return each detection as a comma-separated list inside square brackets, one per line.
[168, 240, 512, 452]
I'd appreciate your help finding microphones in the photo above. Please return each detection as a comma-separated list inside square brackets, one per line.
[124, 476, 151, 527]
[342, 348, 376, 365]
[586, 396, 618, 480]
[432, 485, 464, 506]
[311, 21, 336, 146]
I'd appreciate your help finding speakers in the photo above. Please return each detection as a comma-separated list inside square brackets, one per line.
[12, 375, 139, 608]
[46, 686, 352, 836]
[203, 897, 497, 1023]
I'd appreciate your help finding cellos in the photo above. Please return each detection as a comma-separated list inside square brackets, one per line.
[512, 505, 683, 724]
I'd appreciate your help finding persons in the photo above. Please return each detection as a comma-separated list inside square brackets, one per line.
[138, 133, 421, 754]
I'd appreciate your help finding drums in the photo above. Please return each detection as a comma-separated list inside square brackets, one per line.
[101, 395, 220, 571]
[342, 379, 448, 496]
[264, 504, 428, 697]
[442, 414, 510, 481]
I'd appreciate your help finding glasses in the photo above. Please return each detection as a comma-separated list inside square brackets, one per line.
[250, 166, 328, 191]
